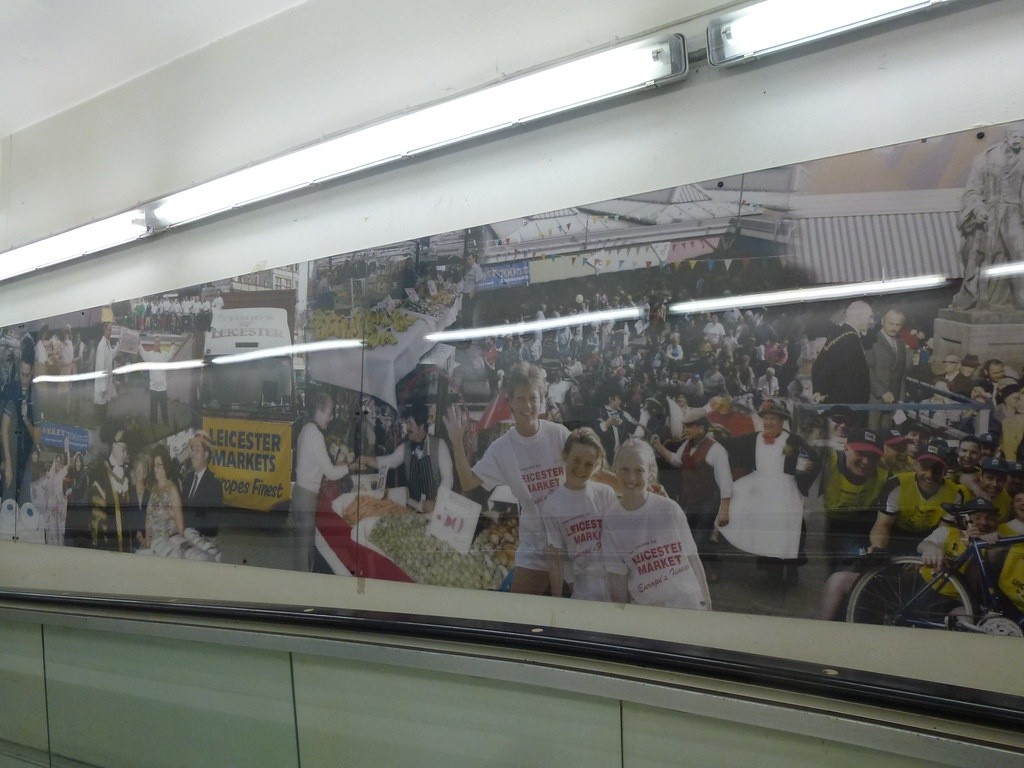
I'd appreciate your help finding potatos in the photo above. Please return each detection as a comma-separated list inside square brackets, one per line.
[364, 512, 521, 589]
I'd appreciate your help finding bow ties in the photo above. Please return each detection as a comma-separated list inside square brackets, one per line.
[763, 432, 781, 444]
[689, 439, 698, 447]
[410, 435, 426, 451]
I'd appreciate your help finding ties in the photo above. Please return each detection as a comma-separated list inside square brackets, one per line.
[188, 474, 198, 502]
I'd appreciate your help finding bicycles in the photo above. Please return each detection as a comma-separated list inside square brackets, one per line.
[840, 501, 1024, 629]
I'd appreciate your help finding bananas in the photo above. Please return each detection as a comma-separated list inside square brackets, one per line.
[309, 306, 417, 349]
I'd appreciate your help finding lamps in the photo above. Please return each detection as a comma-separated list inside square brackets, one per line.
[705, 0, 960, 69]
[0, 31, 689, 286]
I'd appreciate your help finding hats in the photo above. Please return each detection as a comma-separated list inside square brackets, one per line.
[822, 405, 856, 419]
[846, 429, 884, 456]
[915, 443, 949, 467]
[961, 354, 980, 369]
[982, 457, 1007, 475]
[904, 423, 930, 441]
[883, 430, 915, 445]
[757, 398, 790, 419]
[187, 430, 213, 453]
[1008, 461, 1024, 477]
[980, 433, 999, 448]
[680, 410, 707, 424]
[964, 496, 1000, 513]
[938, 446, 959, 465]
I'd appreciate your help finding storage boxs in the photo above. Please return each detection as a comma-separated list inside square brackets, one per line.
[419, 342, 455, 378]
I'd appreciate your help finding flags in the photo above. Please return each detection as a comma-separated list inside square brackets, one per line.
[474, 387, 512, 434]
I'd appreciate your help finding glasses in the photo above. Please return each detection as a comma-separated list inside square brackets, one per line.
[827, 414, 857, 428]
[942, 360, 959, 363]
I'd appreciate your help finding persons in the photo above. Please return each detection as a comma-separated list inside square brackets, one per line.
[348, 402, 452, 512]
[0, 343, 43, 506]
[442, 361, 669, 596]
[652, 408, 732, 551]
[869, 309, 907, 435]
[810, 300, 875, 499]
[600, 439, 712, 611]
[541, 426, 619, 602]
[94, 321, 121, 426]
[139, 337, 173, 425]
[291, 391, 367, 572]
[0, 250, 1024, 640]
[144, 445, 183, 547]
[183, 431, 222, 539]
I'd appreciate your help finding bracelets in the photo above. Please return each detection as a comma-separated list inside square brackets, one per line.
[347, 464, 352, 474]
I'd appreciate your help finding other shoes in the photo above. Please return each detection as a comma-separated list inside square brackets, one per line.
[786, 562, 800, 585]
[709, 556, 721, 581]
[756, 556, 768, 571]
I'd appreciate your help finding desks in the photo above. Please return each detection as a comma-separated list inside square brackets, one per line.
[304, 293, 464, 420]
